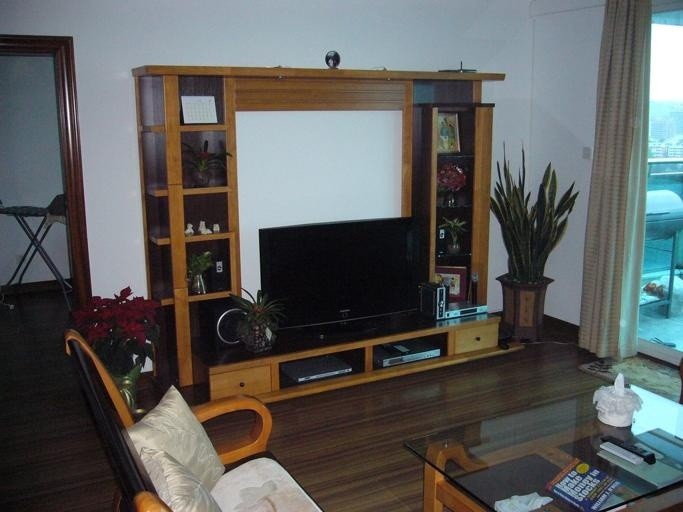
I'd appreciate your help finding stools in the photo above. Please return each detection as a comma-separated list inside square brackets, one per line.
[1, 191, 70, 306]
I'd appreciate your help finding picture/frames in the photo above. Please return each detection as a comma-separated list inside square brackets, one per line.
[435, 111, 461, 155]
[432, 262, 470, 304]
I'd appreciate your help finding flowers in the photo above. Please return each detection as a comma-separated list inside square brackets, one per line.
[53, 285, 163, 358]
[434, 159, 465, 194]
[179, 136, 233, 173]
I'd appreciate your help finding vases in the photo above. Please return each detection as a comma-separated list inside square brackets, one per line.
[441, 190, 457, 210]
[191, 169, 210, 187]
[96, 341, 135, 386]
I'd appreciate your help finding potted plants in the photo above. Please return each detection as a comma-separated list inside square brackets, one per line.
[183, 246, 214, 298]
[437, 211, 467, 257]
[485, 141, 582, 349]
[221, 285, 279, 356]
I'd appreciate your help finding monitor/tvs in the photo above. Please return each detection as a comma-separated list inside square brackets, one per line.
[259, 216, 430, 340]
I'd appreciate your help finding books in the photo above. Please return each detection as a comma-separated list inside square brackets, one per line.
[545, 455, 629, 512]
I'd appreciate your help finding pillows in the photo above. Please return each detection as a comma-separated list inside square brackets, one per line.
[125, 379, 228, 509]
[138, 439, 220, 512]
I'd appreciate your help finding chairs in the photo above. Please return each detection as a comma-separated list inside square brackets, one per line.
[65, 320, 326, 512]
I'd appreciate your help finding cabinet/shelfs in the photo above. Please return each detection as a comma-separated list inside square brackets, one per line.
[188, 302, 525, 410]
[413, 71, 506, 317]
[128, 64, 244, 389]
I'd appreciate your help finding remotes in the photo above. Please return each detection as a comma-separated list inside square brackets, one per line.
[600, 441, 644, 465]
[601, 433, 656, 465]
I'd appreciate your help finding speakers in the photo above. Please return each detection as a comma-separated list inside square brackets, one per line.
[203, 252, 228, 292]
[431, 282, 450, 321]
[436, 227, 447, 256]
[198, 297, 252, 349]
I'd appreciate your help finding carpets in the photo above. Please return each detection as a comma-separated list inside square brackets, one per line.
[576, 353, 681, 403]
